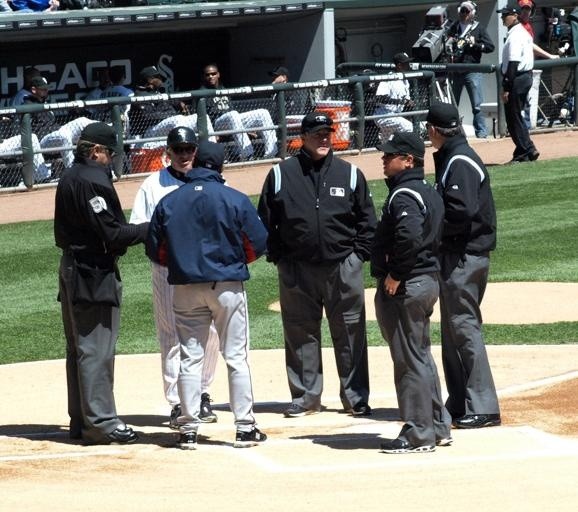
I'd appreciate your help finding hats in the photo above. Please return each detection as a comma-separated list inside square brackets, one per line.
[375, 132, 425, 158]
[269, 65, 289, 75]
[393, 53, 415, 63]
[30, 76, 53, 89]
[496, 3, 522, 13]
[302, 112, 336, 132]
[139, 66, 167, 80]
[422, 103, 459, 127]
[80, 122, 125, 154]
[460, 1, 474, 12]
[195, 141, 225, 169]
[167, 127, 198, 147]
[519, 0, 533, 8]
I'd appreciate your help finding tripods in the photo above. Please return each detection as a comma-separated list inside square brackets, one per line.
[435, 53, 458, 109]
[548, 48, 576, 127]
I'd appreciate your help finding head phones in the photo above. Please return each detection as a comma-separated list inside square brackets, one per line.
[457, 0, 476, 17]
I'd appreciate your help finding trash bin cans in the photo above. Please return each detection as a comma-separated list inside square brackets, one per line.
[524, 68, 543, 129]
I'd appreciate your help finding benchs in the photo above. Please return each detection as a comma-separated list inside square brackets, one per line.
[0, 81, 311, 187]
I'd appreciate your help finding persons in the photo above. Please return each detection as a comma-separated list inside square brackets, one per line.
[1, 65, 305, 187]
[425, 103, 501, 429]
[257, 112, 378, 417]
[129, 126, 219, 429]
[371, 130, 453, 453]
[53, 122, 150, 447]
[375, 0, 495, 144]
[495, 0, 578, 166]
[144, 138, 269, 450]
[1, 0, 204, 14]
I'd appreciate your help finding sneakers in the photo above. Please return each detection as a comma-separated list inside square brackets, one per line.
[176, 432, 198, 450]
[197, 393, 218, 422]
[503, 155, 529, 163]
[168, 404, 181, 429]
[344, 403, 371, 416]
[529, 150, 540, 160]
[241, 156, 262, 162]
[82, 427, 138, 444]
[380, 436, 437, 452]
[282, 404, 321, 417]
[69, 422, 84, 440]
[436, 434, 453, 445]
[264, 154, 279, 165]
[476, 131, 488, 138]
[234, 427, 267, 447]
[452, 415, 500, 428]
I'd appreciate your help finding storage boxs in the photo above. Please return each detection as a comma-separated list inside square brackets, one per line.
[127, 141, 171, 172]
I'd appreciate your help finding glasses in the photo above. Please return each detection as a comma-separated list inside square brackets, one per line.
[206, 72, 216, 76]
[461, 10, 470, 14]
[426, 123, 440, 129]
[106, 149, 117, 159]
[383, 152, 409, 157]
[173, 147, 194, 154]
